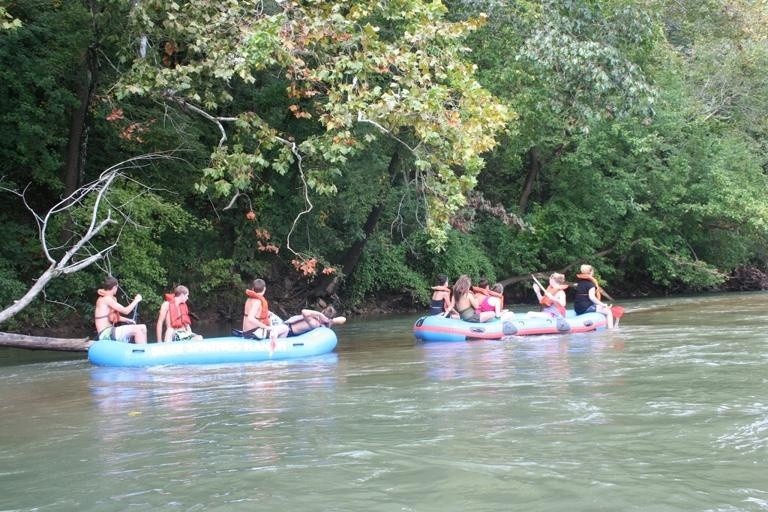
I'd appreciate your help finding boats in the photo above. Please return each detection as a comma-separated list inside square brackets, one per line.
[413, 309, 607, 342]
[88, 327, 339, 368]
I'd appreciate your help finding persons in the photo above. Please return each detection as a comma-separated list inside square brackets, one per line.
[476, 282, 506, 319]
[230, 306, 347, 338]
[94, 277, 148, 347]
[427, 273, 460, 321]
[91, 352, 340, 441]
[443, 275, 496, 325]
[242, 278, 288, 341]
[471, 278, 490, 304]
[572, 263, 617, 332]
[153, 285, 202, 343]
[428, 329, 623, 404]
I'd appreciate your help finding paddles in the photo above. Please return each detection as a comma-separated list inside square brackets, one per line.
[269, 316, 275, 351]
